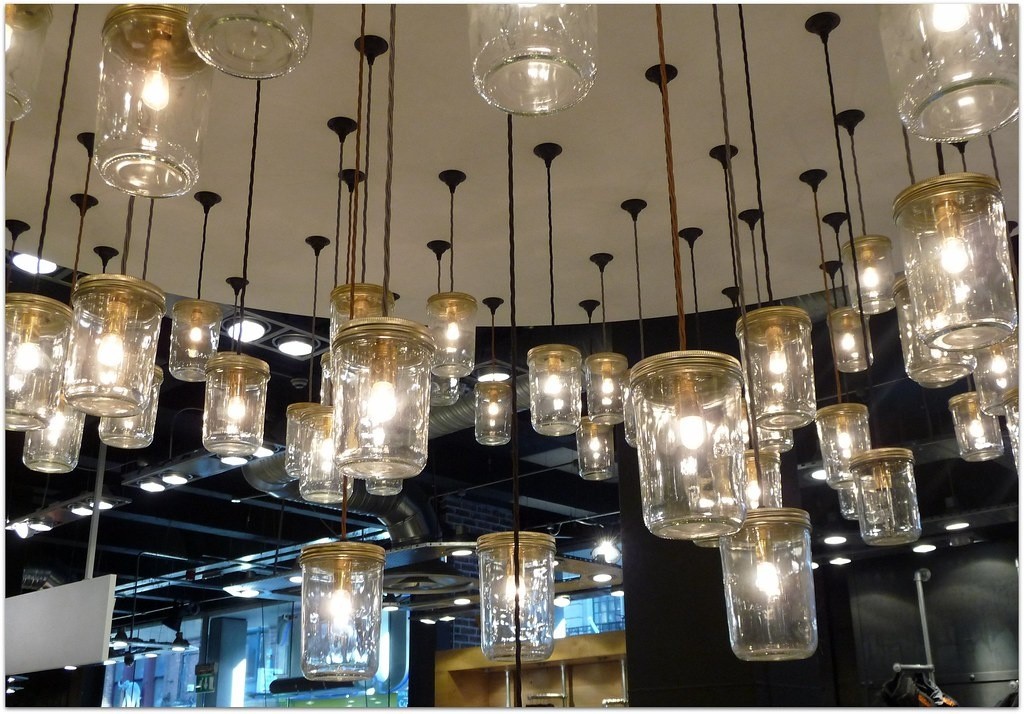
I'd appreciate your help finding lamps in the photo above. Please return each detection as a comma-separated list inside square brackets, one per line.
[890, 117, 1019, 475]
[474, 297, 512, 447]
[882, 4, 1019, 142]
[526, 143, 629, 484]
[5, 463, 131, 539]
[120, 406, 286, 492]
[169, 191, 222, 384]
[468, 4, 600, 117]
[819, 109, 926, 550]
[621, 4, 819, 662]
[285, 4, 478, 682]
[92, 6, 212, 199]
[5, 4, 168, 474]
[475, 92, 559, 665]
[204, 70, 270, 461]
